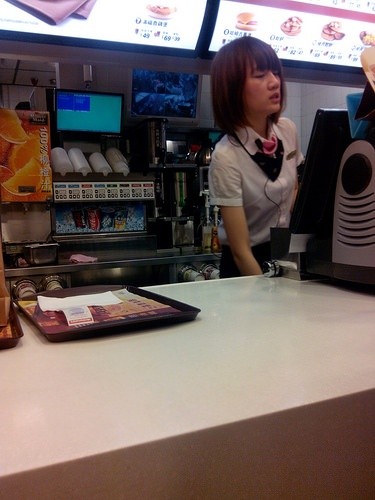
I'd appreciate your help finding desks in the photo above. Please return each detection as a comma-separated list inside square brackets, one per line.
[0, 274, 375, 500]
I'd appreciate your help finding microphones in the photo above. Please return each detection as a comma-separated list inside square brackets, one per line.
[255, 138, 263, 152]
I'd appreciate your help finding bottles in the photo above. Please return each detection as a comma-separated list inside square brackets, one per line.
[89, 152, 112, 176]
[68, 148, 92, 177]
[51, 147, 73, 176]
[106, 148, 128, 173]
[210, 226, 220, 253]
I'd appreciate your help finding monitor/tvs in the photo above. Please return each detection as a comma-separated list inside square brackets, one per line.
[53, 88, 125, 139]
[290, 110, 358, 234]
[109, 63, 203, 125]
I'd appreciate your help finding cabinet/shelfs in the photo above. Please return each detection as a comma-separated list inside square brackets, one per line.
[143, 119, 225, 249]
[50, 115, 156, 247]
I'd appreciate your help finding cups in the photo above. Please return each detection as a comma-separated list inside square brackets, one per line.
[183, 269, 205, 281]
[46, 281, 63, 292]
[16, 283, 36, 298]
[202, 227, 211, 251]
[201, 265, 220, 280]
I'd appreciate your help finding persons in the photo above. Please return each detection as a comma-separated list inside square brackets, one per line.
[207, 36, 304, 280]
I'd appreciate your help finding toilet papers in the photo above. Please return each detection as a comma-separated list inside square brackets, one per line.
[50, 146, 130, 177]
